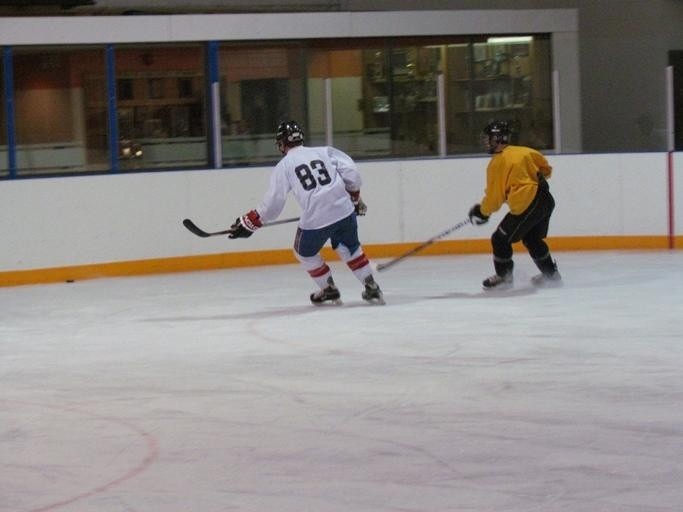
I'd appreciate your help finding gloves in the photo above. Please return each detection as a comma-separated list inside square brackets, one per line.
[358, 197, 367, 216]
[469, 204, 489, 225]
[228, 218, 253, 239]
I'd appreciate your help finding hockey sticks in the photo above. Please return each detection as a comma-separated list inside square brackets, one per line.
[370, 216, 470, 271]
[183, 217, 300, 237]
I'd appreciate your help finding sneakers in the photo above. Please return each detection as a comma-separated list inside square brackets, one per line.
[531, 273, 561, 282]
[361, 283, 382, 300]
[482, 274, 513, 287]
[310, 285, 340, 303]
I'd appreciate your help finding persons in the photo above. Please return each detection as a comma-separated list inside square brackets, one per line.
[468, 121, 565, 291]
[228, 119, 386, 305]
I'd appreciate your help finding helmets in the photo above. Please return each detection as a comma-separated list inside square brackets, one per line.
[480, 120, 511, 153]
[276, 120, 305, 155]
